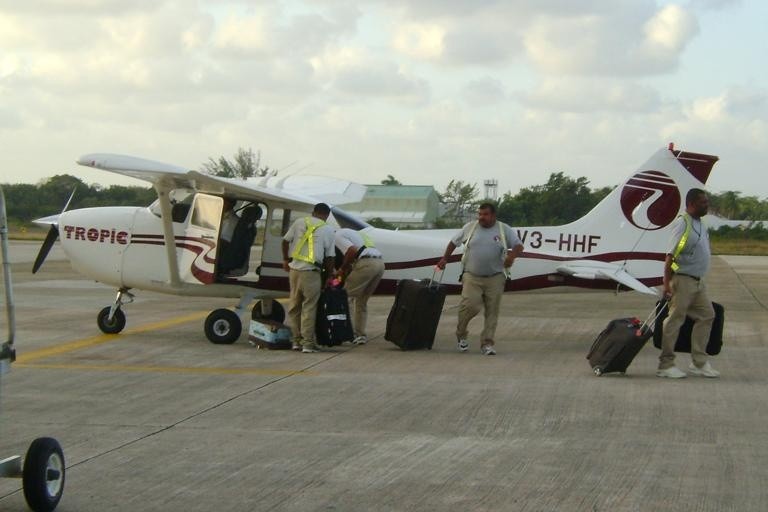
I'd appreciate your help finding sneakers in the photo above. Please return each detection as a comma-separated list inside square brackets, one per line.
[293, 343, 321, 354]
[687, 361, 721, 378]
[341, 335, 368, 347]
[480, 343, 497, 356]
[454, 336, 471, 353]
[655, 366, 687, 379]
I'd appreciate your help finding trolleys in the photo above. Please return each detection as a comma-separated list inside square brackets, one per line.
[0, 185, 66, 512]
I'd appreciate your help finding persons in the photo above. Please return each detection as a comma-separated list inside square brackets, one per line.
[334, 226, 384, 344]
[436, 202, 523, 356]
[655, 187, 722, 378]
[217, 198, 240, 280]
[280, 203, 334, 354]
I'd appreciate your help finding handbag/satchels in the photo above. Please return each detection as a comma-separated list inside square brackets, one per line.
[653, 297, 725, 356]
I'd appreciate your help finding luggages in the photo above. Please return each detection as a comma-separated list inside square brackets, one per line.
[315, 288, 353, 347]
[384, 277, 448, 351]
[586, 317, 653, 377]
[247, 317, 292, 352]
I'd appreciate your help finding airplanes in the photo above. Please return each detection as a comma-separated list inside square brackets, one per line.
[28, 140, 718, 348]
